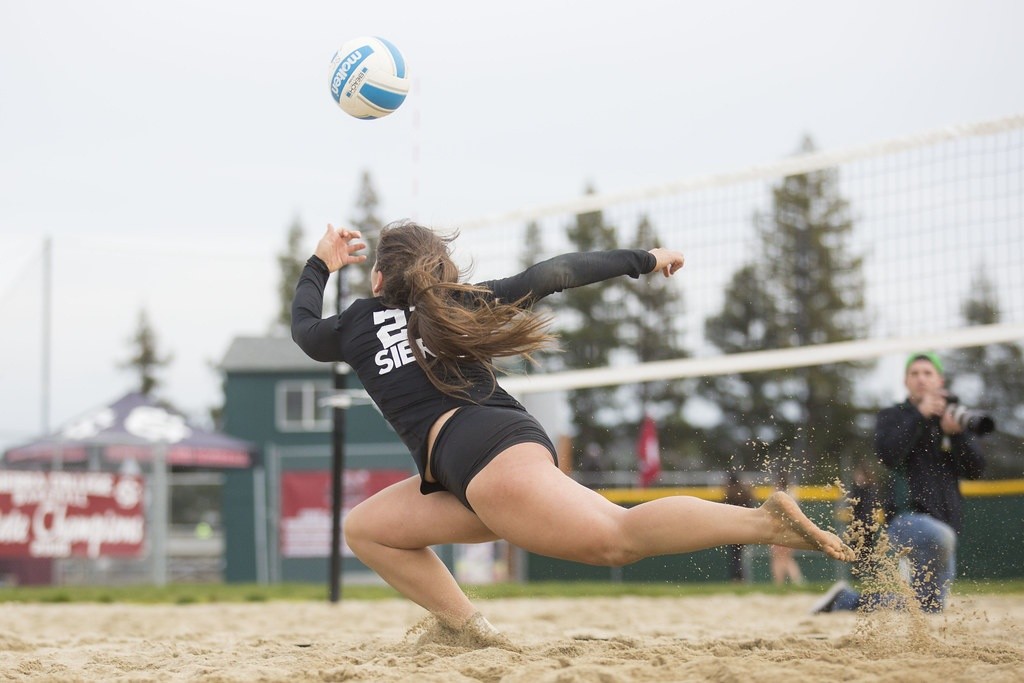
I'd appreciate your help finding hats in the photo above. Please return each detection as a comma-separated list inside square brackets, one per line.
[904, 351, 943, 377]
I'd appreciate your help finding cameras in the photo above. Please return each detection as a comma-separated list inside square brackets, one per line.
[932, 394, 995, 437]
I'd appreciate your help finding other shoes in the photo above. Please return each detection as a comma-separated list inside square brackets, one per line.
[810, 580, 853, 614]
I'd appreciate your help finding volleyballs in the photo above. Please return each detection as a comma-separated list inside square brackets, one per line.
[326, 34, 411, 121]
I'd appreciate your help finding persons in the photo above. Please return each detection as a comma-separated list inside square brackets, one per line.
[815, 356, 983, 613]
[288, 218, 859, 644]
